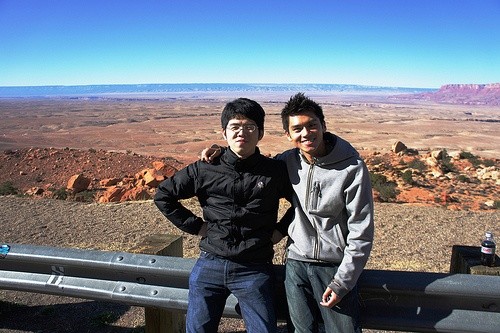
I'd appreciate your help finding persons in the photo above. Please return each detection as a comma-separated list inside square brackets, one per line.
[200, 92, 374, 333]
[154, 98, 295, 333]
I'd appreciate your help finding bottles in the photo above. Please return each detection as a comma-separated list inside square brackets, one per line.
[481, 233, 496, 267]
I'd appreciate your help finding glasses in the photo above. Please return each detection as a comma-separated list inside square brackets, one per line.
[226, 124, 259, 133]
